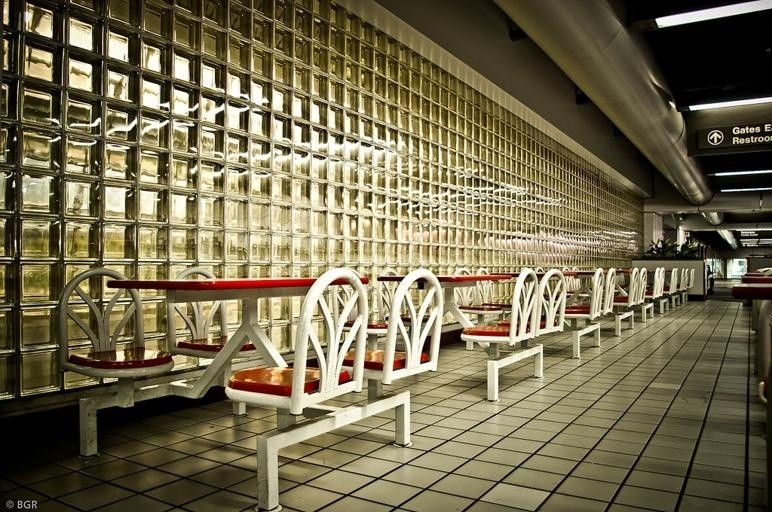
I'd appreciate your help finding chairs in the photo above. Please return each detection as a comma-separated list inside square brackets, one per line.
[55, 267, 694, 511]
